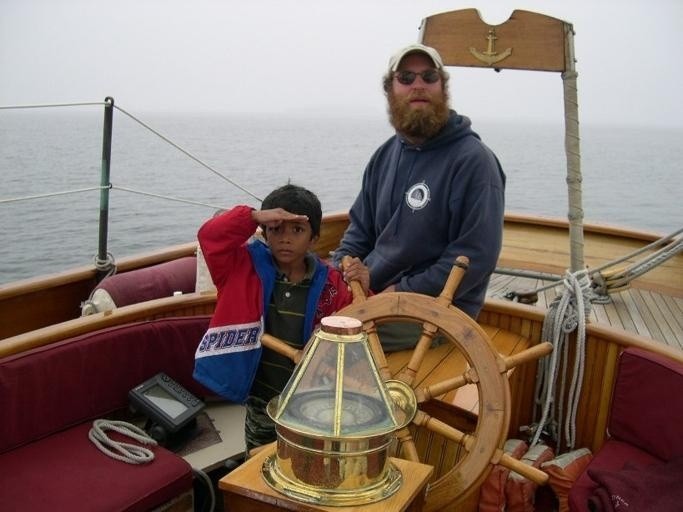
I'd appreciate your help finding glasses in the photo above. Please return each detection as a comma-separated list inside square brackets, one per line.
[391, 69, 443, 84]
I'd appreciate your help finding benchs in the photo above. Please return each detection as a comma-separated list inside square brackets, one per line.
[0, 315, 214, 510]
[569, 346, 682, 511]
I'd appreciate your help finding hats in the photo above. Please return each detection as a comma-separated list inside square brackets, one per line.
[388, 43, 444, 71]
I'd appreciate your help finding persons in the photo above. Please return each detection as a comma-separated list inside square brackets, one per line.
[191, 180, 369, 470]
[322, 41, 508, 370]
